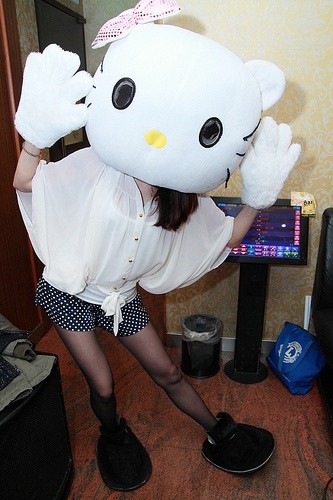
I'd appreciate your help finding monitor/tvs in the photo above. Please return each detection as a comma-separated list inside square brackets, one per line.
[210, 197, 309, 265]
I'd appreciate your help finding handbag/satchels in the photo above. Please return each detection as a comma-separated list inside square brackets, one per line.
[267, 322, 326, 395]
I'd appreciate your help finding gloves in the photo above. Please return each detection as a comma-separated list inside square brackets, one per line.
[15, 43, 94, 150]
[238, 115, 302, 210]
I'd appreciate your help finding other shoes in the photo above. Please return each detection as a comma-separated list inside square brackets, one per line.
[201, 410, 276, 475]
[96, 418, 154, 491]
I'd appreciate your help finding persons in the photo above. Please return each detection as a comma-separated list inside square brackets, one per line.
[13, 0, 302, 491]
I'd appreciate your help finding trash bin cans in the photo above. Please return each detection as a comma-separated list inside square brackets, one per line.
[179, 313, 224, 379]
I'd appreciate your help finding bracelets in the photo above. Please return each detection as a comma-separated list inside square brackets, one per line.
[22, 141, 42, 158]
